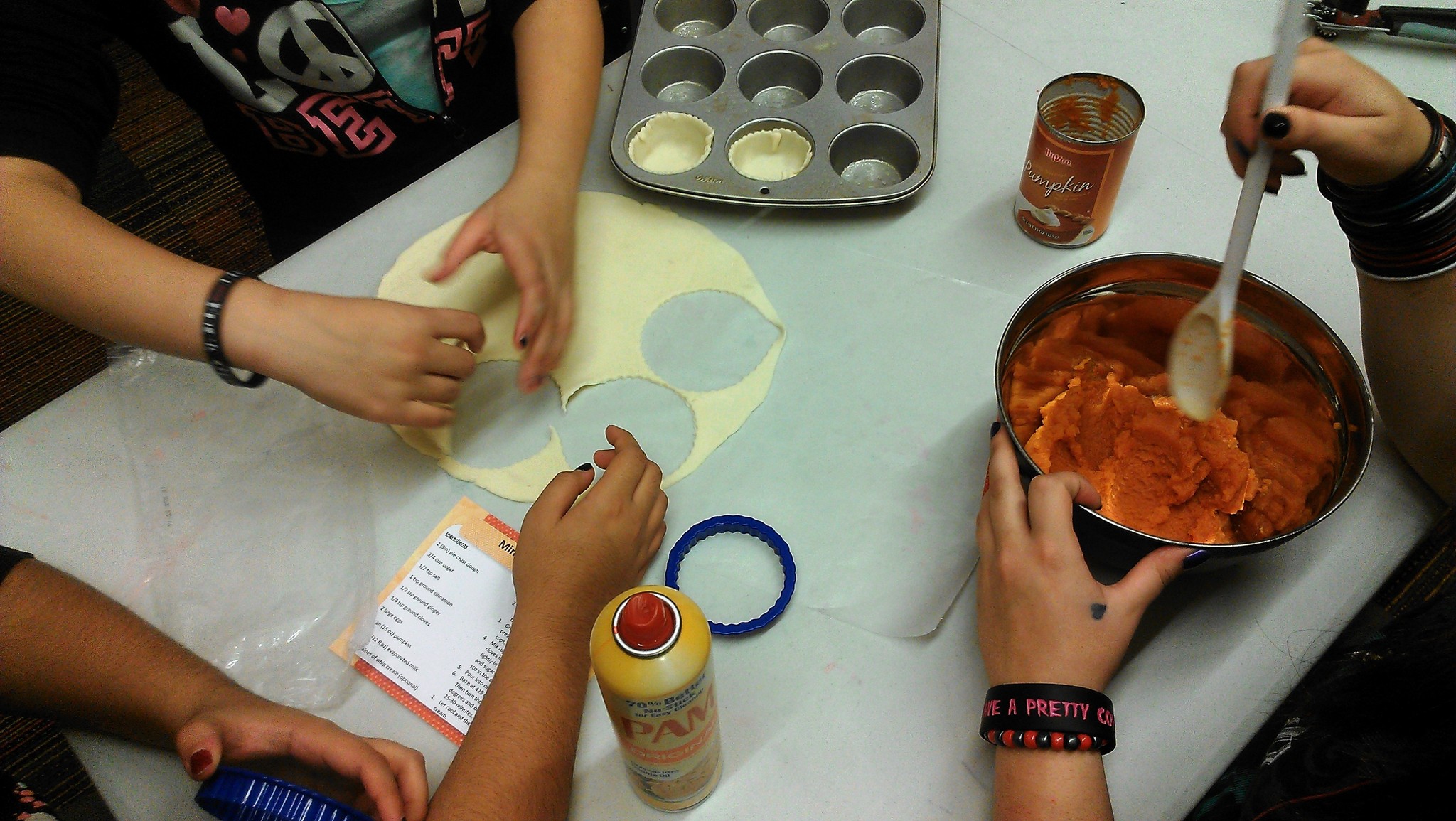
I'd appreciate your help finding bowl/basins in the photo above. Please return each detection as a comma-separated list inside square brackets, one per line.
[994, 251, 1375, 561]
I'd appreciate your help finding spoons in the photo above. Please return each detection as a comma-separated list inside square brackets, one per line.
[1169, 14, 1296, 421]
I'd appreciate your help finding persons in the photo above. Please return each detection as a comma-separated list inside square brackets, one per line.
[977, 34, 1456, 821]
[0, 0, 604, 428]
[0, 424, 668, 820]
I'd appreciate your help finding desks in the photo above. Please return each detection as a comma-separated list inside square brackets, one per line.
[0, 0, 1456, 821]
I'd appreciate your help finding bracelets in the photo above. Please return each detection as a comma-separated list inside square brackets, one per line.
[201, 267, 268, 389]
[979, 679, 1116, 756]
[1317, 98, 1456, 281]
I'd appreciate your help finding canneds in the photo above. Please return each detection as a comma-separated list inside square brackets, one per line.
[1014, 72, 1146, 250]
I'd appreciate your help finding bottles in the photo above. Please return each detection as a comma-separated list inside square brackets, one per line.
[589, 585, 722, 812]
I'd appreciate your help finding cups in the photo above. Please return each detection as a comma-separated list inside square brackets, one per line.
[1013, 71, 1146, 249]
